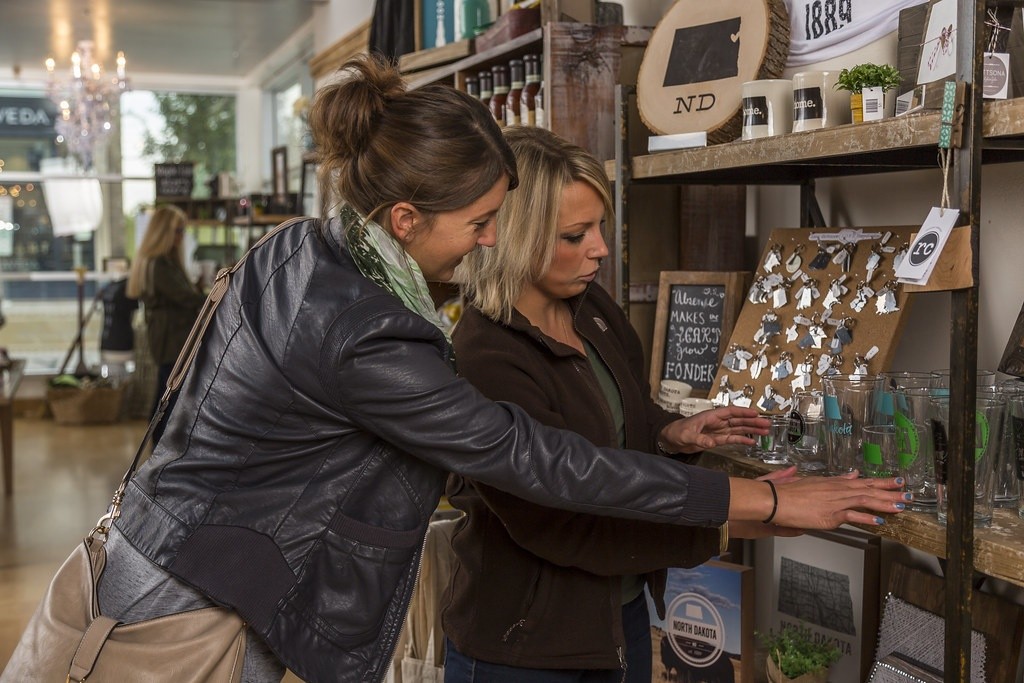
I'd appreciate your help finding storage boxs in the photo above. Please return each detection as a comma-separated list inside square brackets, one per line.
[44, 375, 121, 423]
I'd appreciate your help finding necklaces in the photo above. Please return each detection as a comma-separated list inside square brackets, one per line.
[558, 307, 568, 343]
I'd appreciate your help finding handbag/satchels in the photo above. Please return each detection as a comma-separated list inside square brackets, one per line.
[0, 527, 246, 683]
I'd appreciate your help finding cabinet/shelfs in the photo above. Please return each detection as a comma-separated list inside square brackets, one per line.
[612, 84, 1024, 683]
[404, 17, 658, 300]
[152, 193, 299, 267]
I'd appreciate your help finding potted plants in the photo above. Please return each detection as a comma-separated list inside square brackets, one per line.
[750, 621, 844, 683]
[839, 62, 900, 124]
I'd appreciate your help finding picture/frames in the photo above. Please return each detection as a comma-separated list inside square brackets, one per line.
[752, 525, 944, 683]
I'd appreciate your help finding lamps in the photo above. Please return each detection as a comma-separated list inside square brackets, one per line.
[44, 40, 131, 170]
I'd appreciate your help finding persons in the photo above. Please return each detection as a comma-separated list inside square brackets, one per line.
[96, 50, 912, 683]
[441, 124, 800, 683]
[127, 206, 213, 454]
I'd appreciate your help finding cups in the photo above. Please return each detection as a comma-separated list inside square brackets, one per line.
[791, 69, 852, 132]
[739, 365, 1024, 529]
[740, 76, 794, 141]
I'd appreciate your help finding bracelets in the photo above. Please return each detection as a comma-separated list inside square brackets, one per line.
[720, 521, 728, 552]
[658, 442, 680, 456]
[763, 479, 777, 524]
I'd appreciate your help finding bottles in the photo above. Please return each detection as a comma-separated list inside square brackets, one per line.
[465, 53, 545, 133]
